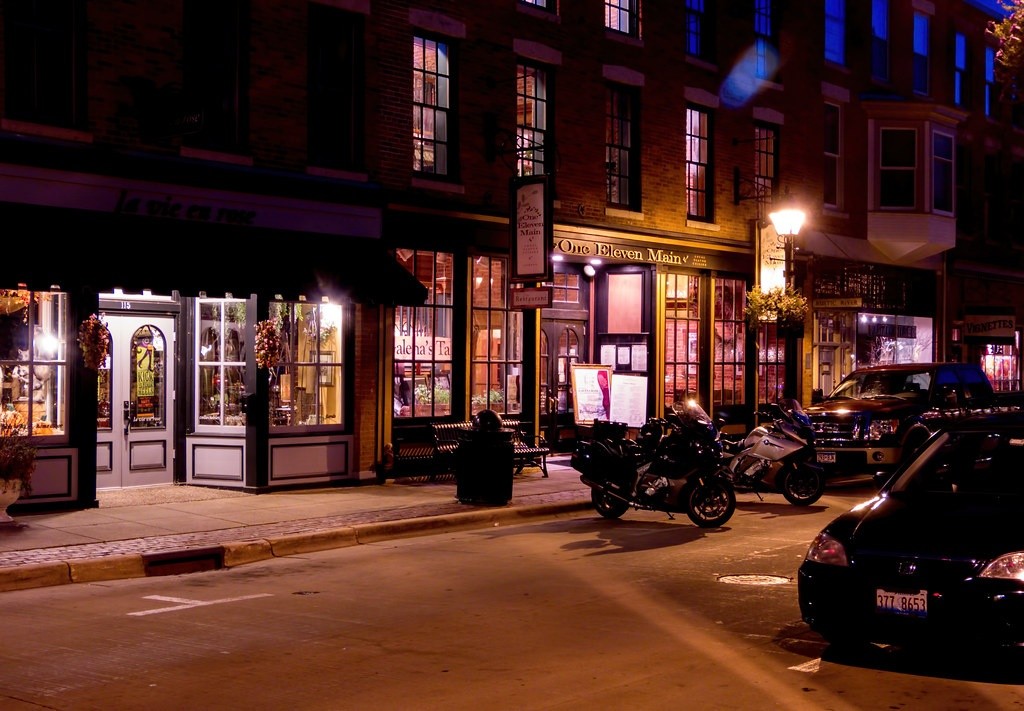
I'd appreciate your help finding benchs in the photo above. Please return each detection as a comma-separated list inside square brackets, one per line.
[427, 417, 550, 485]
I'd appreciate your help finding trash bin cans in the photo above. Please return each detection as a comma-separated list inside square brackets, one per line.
[454, 429, 517, 505]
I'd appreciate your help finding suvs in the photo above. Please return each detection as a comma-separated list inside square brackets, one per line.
[800, 362, 997, 489]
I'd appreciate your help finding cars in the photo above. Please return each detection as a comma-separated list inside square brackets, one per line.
[797, 411, 1024, 643]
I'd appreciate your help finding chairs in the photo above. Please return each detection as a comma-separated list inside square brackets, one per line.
[903, 382, 927, 393]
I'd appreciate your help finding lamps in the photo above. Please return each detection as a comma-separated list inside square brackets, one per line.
[583, 263, 599, 277]
[395, 246, 415, 263]
[476, 256, 489, 268]
[436, 251, 448, 265]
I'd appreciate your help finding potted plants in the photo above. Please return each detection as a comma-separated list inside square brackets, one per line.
[743, 283, 808, 337]
[0, 417, 44, 523]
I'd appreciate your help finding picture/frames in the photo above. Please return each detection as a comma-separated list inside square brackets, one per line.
[570, 363, 613, 425]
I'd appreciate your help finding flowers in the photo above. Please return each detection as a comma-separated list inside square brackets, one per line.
[254, 316, 283, 368]
[75, 313, 109, 370]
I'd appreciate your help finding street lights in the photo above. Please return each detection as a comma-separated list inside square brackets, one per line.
[767, 185, 808, 397]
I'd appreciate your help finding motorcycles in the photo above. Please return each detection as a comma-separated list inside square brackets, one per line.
[706, 398, 825, 506]
[570, 388, 736, 528]
